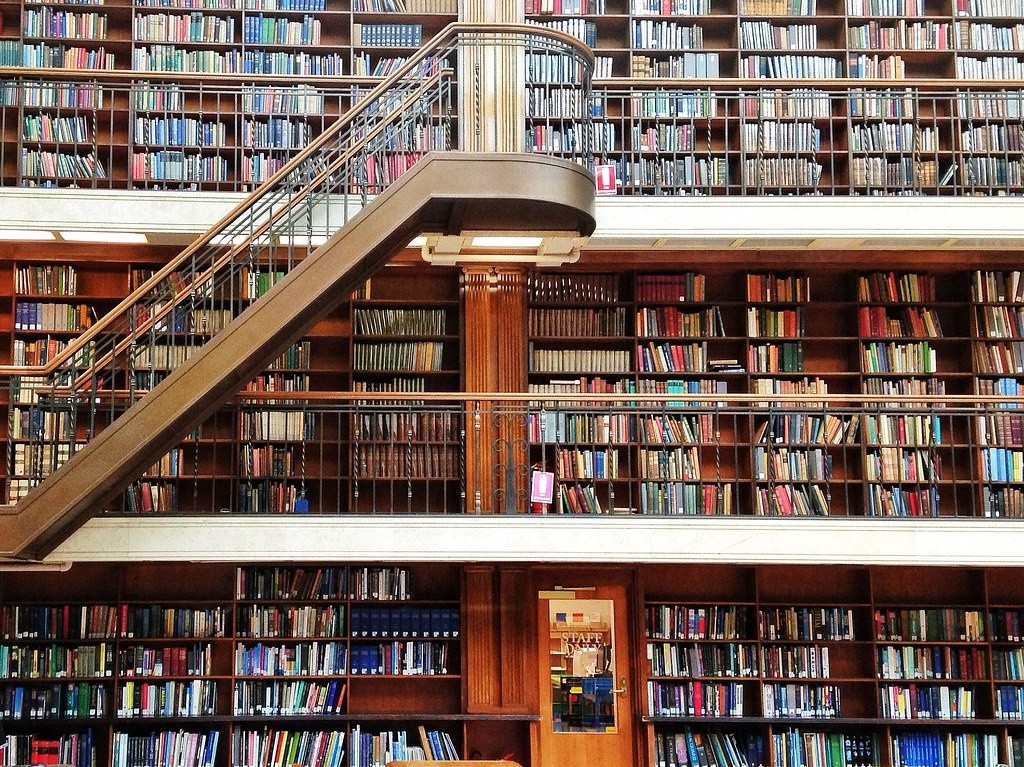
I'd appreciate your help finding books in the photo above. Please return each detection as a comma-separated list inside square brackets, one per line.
[9, 266, 1024, 518]
[0, 566, 1023, 767]
[0, 0, 1024, 197]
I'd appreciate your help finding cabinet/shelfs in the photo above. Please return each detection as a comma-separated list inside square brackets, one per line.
[1, 1, 1024, 767]
